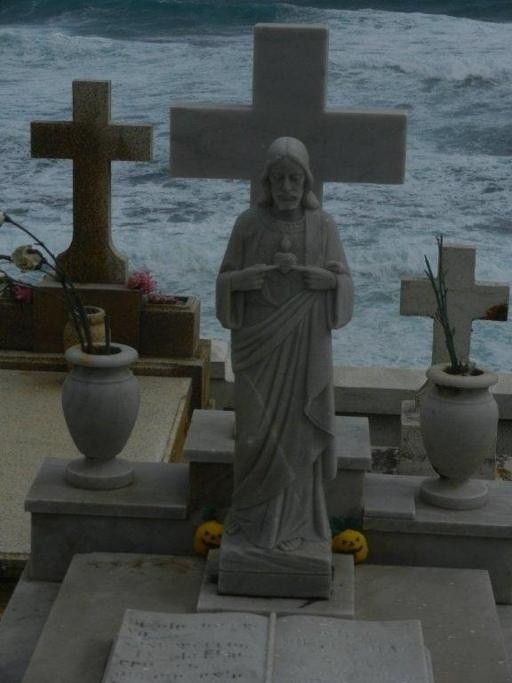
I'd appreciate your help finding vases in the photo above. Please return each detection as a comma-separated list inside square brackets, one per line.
[59, 301, 114, 360]
[414, 359, 500, 512]
[50, 334, 146, 491]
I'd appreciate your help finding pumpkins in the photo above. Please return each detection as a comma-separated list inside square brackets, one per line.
[332, 528, 369, 564]
[193, 519, 224, 556]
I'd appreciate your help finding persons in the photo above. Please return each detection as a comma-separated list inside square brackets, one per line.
[213, 136, 354, 553]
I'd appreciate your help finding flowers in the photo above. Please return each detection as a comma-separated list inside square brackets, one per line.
[125, 263, 181, 307]
[8, 282, 35, 303]
